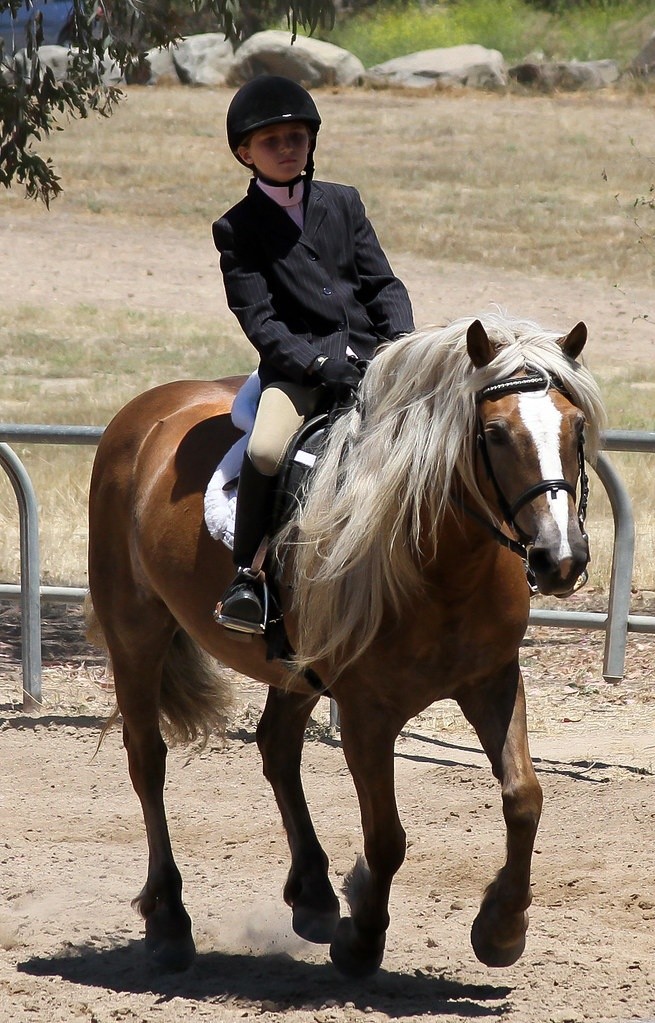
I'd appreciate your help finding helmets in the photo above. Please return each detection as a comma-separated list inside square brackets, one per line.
[226, 76, 321, 170]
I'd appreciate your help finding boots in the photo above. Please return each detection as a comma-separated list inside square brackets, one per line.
[220, 448, 282, 644]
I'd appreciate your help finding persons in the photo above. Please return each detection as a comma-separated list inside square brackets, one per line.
[213, 76, 416, 635]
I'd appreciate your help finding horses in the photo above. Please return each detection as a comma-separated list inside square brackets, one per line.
[87, 315, 605, 983]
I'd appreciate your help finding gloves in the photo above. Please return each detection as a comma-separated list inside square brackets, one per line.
[308, 354, 362, 401]
